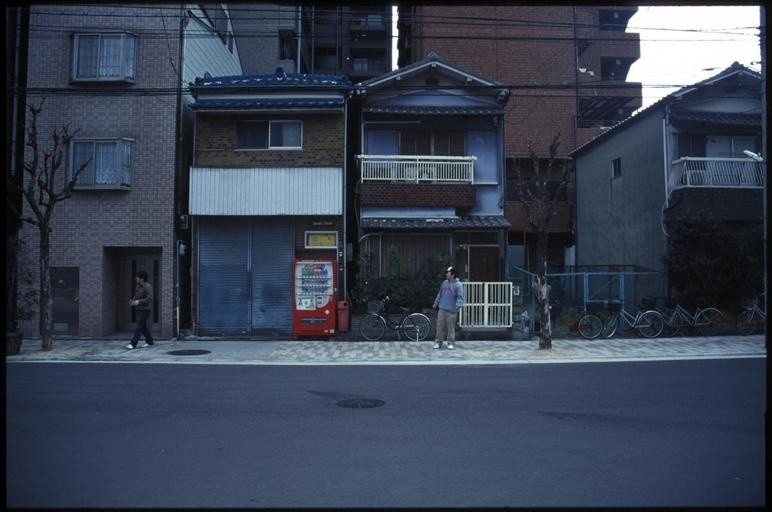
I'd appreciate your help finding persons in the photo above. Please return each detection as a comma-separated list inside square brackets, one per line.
[125, 271, 154, 350]
[432, 267, 465, 351]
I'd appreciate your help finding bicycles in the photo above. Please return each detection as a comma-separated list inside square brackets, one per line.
[358, 296, 433, 345]
[550, 292, 767, 340]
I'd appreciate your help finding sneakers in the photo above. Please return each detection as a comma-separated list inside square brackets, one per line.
[433, 342, 441, 349]
[141, 343, 149, 348]
[447, 344, 454, 349]
[126, 344, 133, 350]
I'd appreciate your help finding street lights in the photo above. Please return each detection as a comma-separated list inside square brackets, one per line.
[173, 237, 187, 338]
[742, 148, 764, 164]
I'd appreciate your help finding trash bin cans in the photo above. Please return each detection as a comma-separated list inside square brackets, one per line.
[337, 301, 353, 334]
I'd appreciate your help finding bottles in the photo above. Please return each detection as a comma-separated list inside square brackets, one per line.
[302, 267, 329, 295]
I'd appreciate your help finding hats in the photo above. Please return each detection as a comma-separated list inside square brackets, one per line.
[443, 266, 456, 272]
